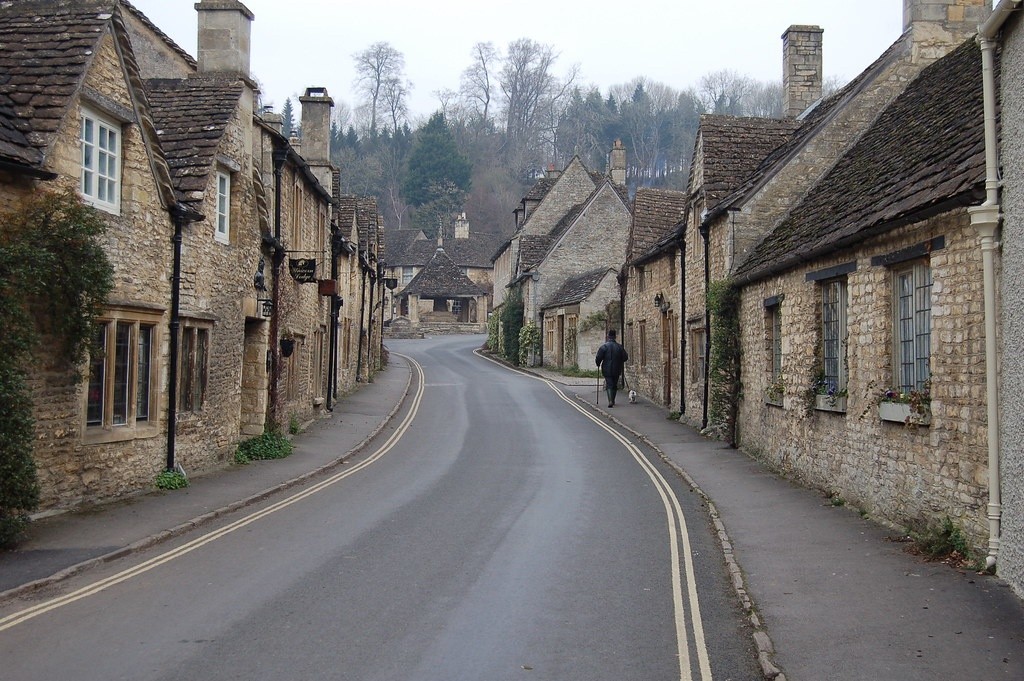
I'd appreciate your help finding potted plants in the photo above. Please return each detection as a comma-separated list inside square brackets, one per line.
[279, 328, 294, 358]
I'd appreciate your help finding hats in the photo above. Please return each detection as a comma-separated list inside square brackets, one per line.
[608, 330, 616, 340]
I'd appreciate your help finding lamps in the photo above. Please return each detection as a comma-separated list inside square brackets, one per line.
[384, 295, 389, 305]
[256, 297, 274, 318]
[654, 292, 663, 308]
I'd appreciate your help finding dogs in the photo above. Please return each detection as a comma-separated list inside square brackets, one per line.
[628, 389, 636, 403]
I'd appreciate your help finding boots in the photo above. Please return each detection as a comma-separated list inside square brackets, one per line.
[613, 388, 616, 404]
[607, 388, 614, 407]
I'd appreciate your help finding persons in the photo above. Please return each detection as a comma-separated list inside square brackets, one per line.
[595, 329, 628, 408]
